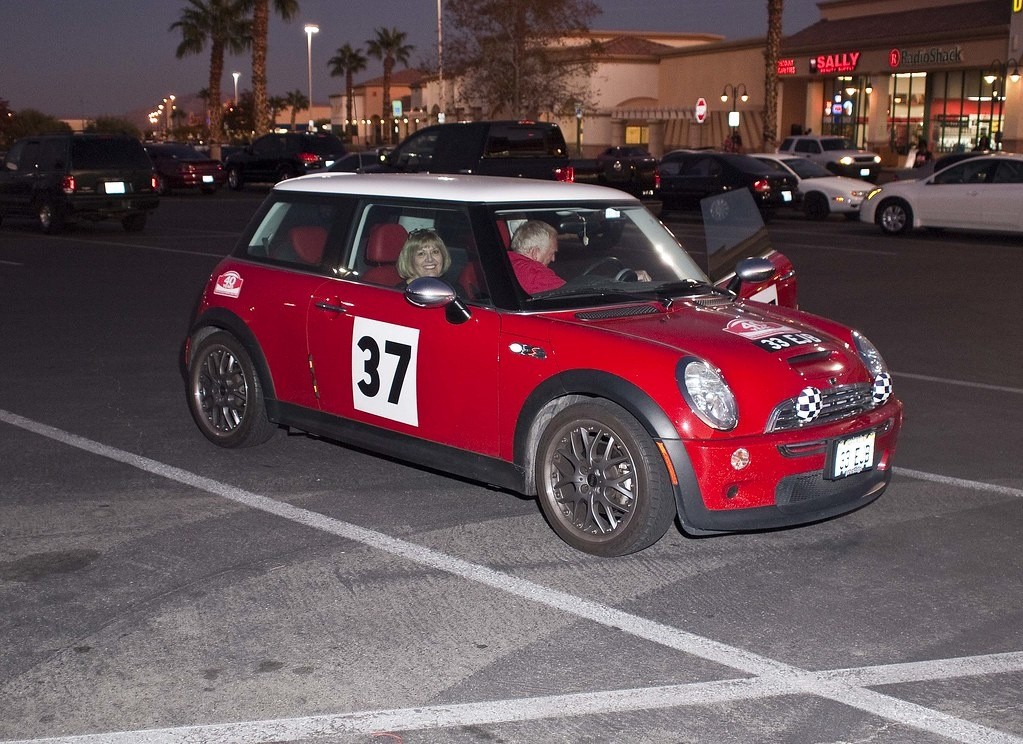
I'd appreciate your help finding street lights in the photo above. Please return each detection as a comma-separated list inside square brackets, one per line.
[845, 72, 873, 150]
[983, 58, 1021, 151]
[345, 115, 419, 150]
[149, 95, 176, 139]
[232, 73, 240, 108]
[721, 83, 749, 154]
[305, 24, 319, 132]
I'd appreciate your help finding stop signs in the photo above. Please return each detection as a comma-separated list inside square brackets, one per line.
[695, 97, 707, 123]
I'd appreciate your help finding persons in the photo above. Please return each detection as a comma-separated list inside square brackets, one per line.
[508, 219, 652, 302]
[396, 230, 463, 297]
[972, 136, 993, 151]
[724, 131, 743, 152]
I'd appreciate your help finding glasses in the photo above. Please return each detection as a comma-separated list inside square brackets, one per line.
[408, 227, 436, 241]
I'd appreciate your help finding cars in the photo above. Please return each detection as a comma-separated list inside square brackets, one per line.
[860, 153, 1022, 238]
[322, 120, 804, 225]
[747, 154, 877, 222]
[184, 172, 904, 558]
[144, 144, 229, 198]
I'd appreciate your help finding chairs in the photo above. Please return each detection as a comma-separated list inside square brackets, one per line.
[290, 223, 328, 265]
[359, 221, 409, 287]
[460, 220, 512, 302]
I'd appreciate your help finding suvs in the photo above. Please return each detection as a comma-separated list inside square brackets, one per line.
[223, 130, 347, 192]
[775, 136, 880, 183]
[0, 128, 160, 234]
